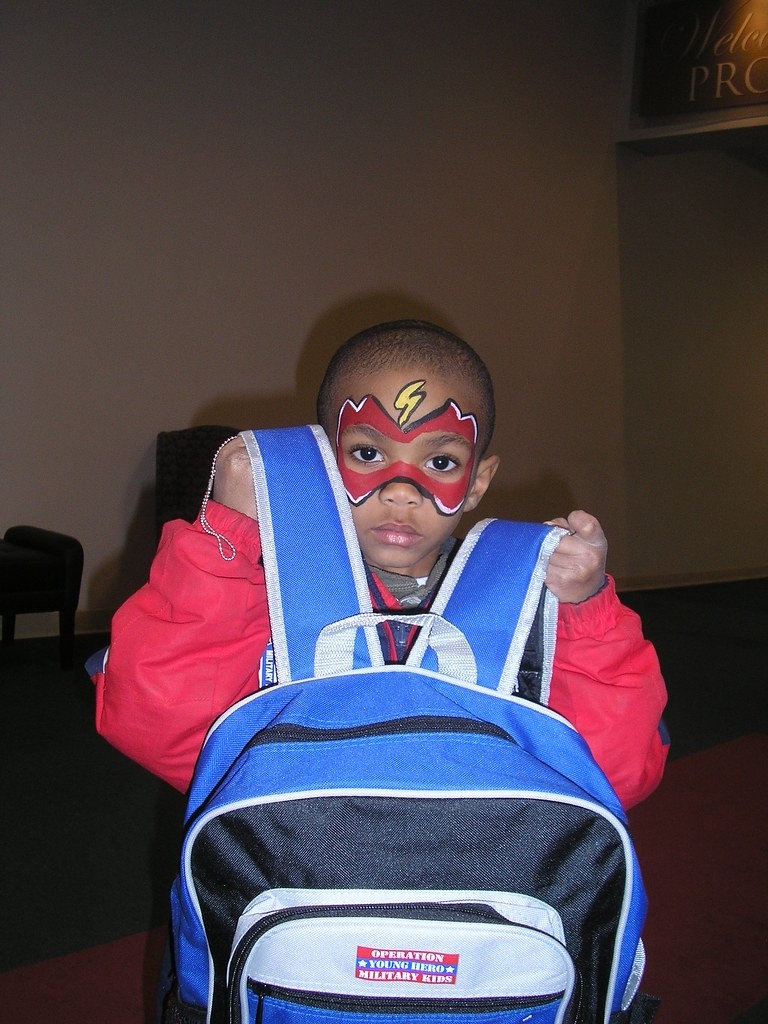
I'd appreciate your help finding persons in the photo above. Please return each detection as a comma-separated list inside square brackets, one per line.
[83, 320, 671, 815]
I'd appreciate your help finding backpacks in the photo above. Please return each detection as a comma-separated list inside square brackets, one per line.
[158, 422, 647, 1024]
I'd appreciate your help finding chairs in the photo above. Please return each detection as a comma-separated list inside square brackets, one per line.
[150, 426, 247, 543]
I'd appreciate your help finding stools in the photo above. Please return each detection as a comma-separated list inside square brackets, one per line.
[0, 523, 85, 674]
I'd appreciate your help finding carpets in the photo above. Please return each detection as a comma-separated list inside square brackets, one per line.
[0, 729, 768, 1024]
